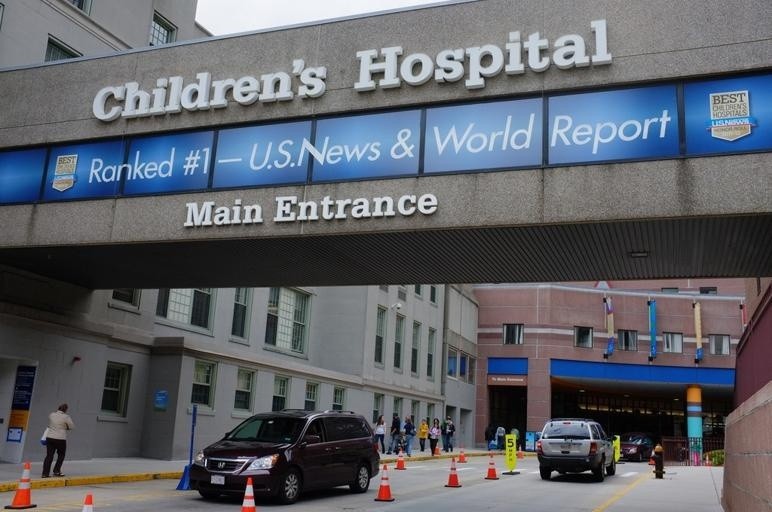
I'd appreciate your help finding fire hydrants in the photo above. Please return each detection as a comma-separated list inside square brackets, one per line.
[650, 441, 666, 479]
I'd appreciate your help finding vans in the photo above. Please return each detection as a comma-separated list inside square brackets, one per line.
[187, 407, 382, 506]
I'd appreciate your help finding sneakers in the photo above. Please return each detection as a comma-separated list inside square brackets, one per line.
[41, 472, 51, 478]
[53, 472, 65, 476]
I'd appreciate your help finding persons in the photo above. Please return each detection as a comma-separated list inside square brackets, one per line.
[374, 415, 385, 454]
[41, 403, 74, 478]
[445, 415, 455, 452]
[386, 413, 400, 455]
[404, 419, 417, 457]
[401, 416, 410, 431]
[496, 426, 505, 450]
[511, 425, 520, 449]
[485, 424, 496, 444]
[428, 418, 441, 456]
[441, 421, 445, 451]
[416, 418, 429, 451]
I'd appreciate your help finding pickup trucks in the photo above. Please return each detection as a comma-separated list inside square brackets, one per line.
[661, 426, 722, 461]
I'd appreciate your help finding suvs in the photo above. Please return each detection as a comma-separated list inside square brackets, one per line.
[534, 416, 618, 482]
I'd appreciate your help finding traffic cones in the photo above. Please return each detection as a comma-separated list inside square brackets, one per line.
[441, 457, 463, 489]
[375, 463, 395, 503]
[484, 455, 500, 481]
[5, 460, 39, 510]
[240, 476, 258, 512]
[394, 445, 407, 473]
[82, 492, 95, 512]
[457, 449, 467, 464]
[433, 441, 440, 459]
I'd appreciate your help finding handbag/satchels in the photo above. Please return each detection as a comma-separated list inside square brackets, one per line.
[40, 427, 50, 445]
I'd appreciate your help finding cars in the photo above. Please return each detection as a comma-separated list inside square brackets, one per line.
[616, 431, 651, 462]
